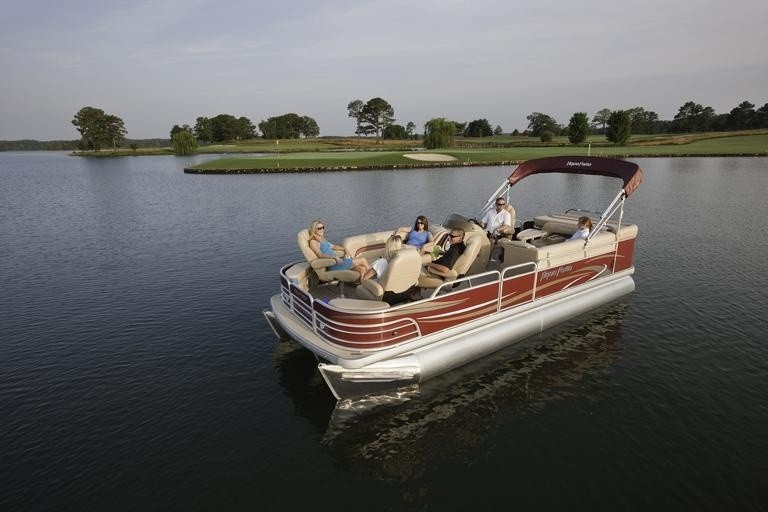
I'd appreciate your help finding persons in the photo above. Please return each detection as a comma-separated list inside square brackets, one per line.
[417, 228, 466, 289]
[563, 217, 592, 239]
[395, 215, 434, 254]
[361, 236, 402, 281]
[308, 221, 370, 280]
[480, 198, 512, 262]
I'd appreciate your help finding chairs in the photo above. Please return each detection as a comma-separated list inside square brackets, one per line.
[297, 224, 483, 301]
[504, 204, 517, 240]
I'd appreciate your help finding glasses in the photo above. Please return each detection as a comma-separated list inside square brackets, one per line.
[449, 233, 459, 238]
[498, 203, 505, 205]
[417, 221, 424, 224]
[317, 225, 325, 230]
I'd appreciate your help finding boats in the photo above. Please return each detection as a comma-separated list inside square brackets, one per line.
[262, 155, 644, 402]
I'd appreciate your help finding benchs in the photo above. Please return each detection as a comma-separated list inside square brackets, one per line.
[496, 222, 617, 270]
